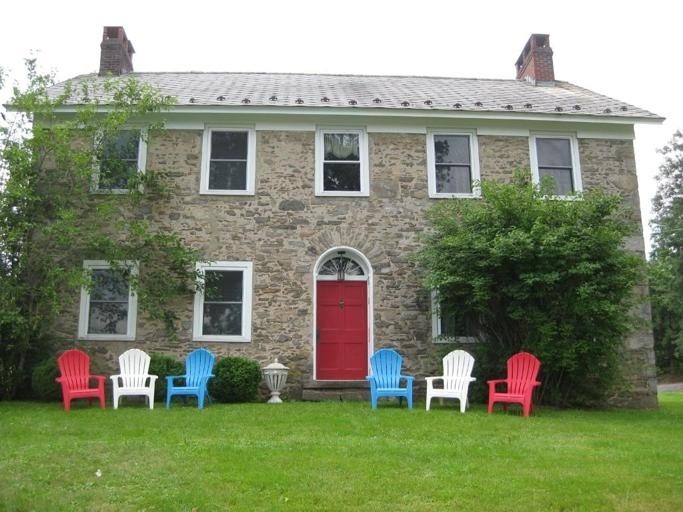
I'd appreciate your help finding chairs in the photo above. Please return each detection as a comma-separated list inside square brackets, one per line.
[425, 350, 477, 413]
[55, 350, 106, 411]
[166, 348, 216, 410]
[110, 349, 159, 410]
[487, 352, 542, 416]
[366, 349, 416, 410]
